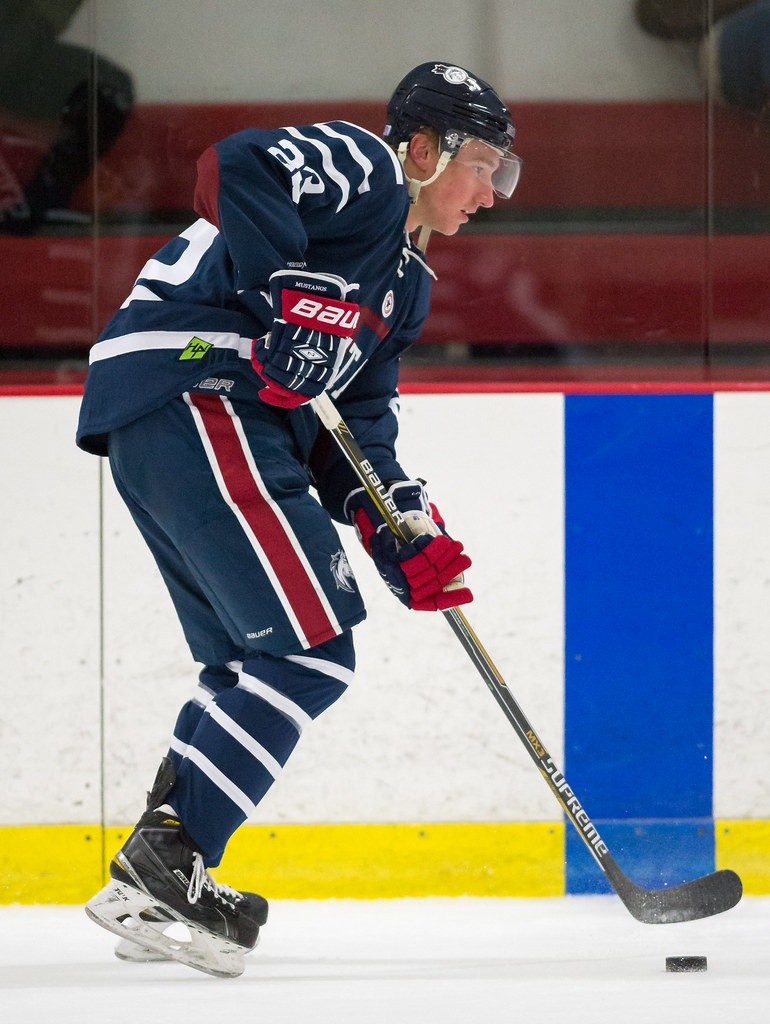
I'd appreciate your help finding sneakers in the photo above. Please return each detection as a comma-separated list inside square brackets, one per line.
[85, 757, 268, 978]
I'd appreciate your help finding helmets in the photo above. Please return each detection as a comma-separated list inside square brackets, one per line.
[383, 62, 516, 161]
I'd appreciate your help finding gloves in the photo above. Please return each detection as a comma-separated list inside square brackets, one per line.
[251, 270, 361, 409]
[344, 477, 474, 611]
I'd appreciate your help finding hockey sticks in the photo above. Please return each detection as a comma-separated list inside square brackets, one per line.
[310, 393, 744, 925]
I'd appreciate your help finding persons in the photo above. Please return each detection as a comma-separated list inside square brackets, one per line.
[76, 63, 520, 979]
[637, 0, 770, 118]
[1, 0, 139, 235]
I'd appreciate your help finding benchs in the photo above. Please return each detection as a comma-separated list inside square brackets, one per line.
[0, 95, 770, 383]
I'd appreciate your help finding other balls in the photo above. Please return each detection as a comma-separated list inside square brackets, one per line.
[666, 955, 708, 972]
